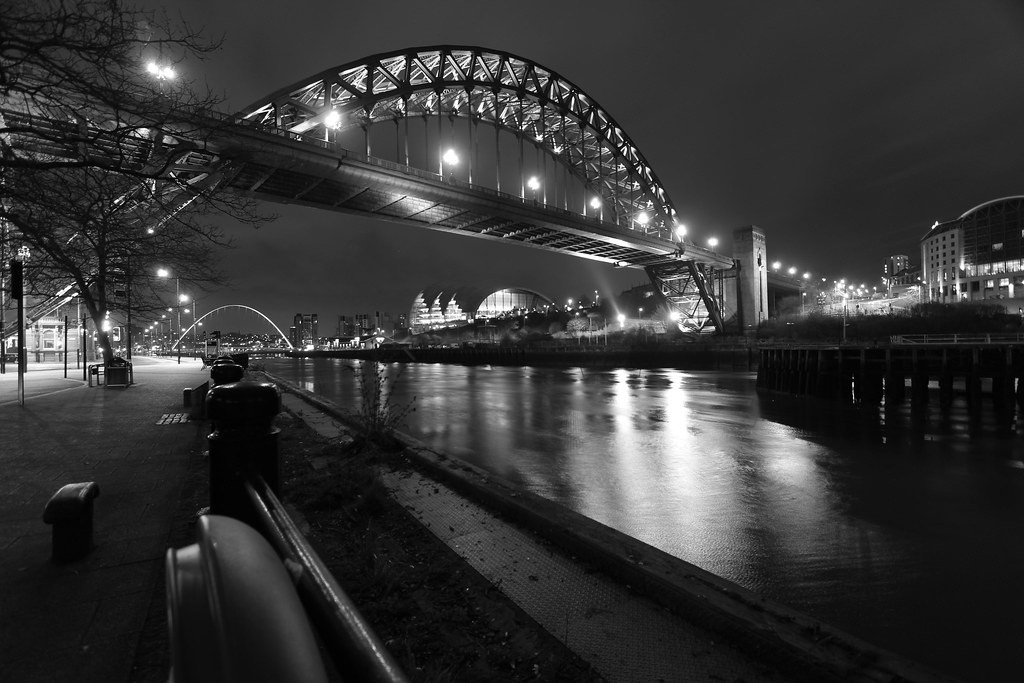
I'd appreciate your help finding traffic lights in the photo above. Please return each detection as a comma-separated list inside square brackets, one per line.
[113, 327, 120, 342]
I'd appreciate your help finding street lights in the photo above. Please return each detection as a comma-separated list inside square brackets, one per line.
[145, 294, 202, 360]
[158, 269, 181, 363]
